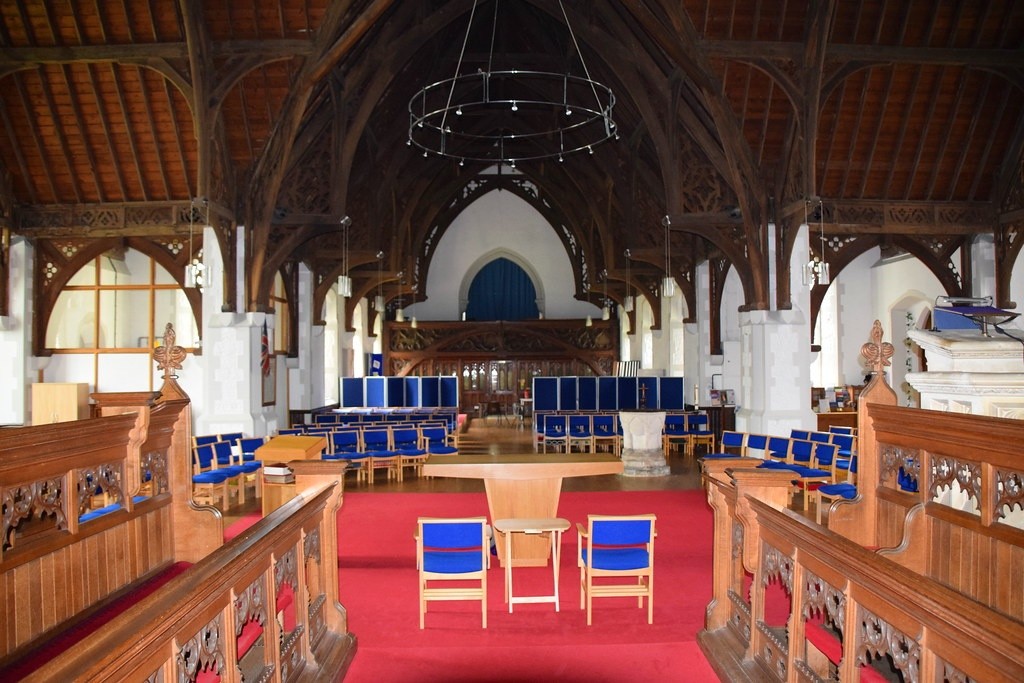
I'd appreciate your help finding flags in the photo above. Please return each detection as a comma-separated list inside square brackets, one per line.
[261, 318, 271, 376]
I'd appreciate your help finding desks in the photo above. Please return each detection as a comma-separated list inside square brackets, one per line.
[420, 454, 625, 568]
[812, 407, 858, 436]
[477, 400, 513, 428]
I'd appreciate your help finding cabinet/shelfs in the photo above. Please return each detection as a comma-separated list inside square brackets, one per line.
[30, 382, 91, 425]
[699, 407, 738, 455]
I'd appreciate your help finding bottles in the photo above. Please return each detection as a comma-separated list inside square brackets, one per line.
[525, 390, 529, 399]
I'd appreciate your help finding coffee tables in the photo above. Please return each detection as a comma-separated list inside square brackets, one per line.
[493, 517, 572, 613]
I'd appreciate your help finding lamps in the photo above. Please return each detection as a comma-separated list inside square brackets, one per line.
[662, 215, 675, 299]
[184, 197, 213, 288]
[586, 273, 592, 326]
[337, 192, 417, 329]
[602, 268, 609, 321]
[623, 248, 634, 313]
[801, 199, 830, 286]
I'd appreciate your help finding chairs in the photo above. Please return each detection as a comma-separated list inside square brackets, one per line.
[535, 413, 922, 524]
[411, 516, 492, 631]
[509, 399, 532, 432]
[77, 408, 459, 530]
[577, 513, 658, 626]
